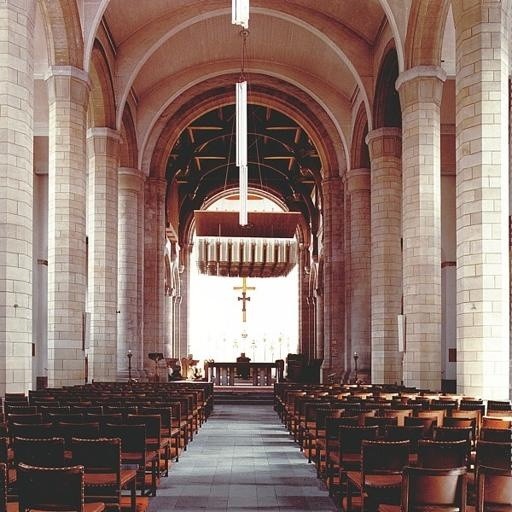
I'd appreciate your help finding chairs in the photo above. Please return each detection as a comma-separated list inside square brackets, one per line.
[274, 381, 511, 512]
[0, 383, 213, 511]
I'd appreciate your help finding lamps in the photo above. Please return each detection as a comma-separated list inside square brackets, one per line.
[237, 166, 248, 226]
[192, 99, 303, 281]
[231, 0, 255, 28]
[234, 34, 247, 164]
